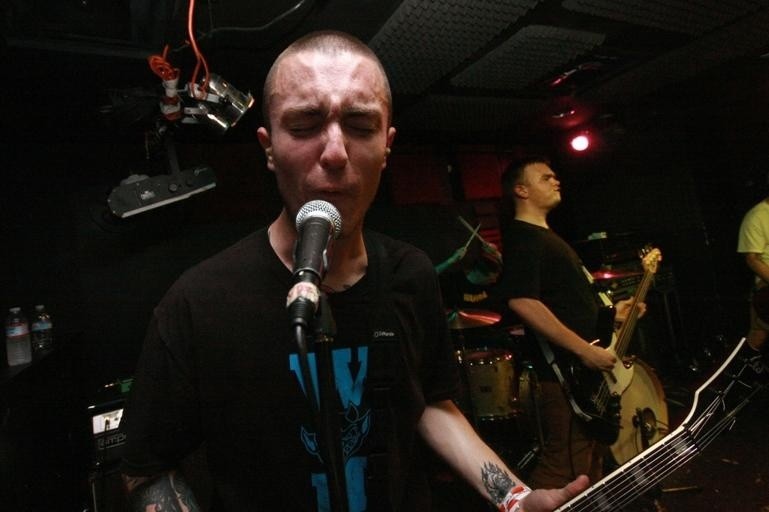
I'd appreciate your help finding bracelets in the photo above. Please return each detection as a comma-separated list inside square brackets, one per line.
[498, 484, 532, 512]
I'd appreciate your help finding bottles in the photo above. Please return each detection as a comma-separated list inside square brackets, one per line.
[30, 302, 54, 352]
[4, 304, 33, 368]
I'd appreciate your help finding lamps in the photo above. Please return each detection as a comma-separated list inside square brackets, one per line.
[192, 70, 255, 134]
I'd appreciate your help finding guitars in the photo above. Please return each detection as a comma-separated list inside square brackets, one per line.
[558, 245, 662, 424]
[553, 337, 769, 511]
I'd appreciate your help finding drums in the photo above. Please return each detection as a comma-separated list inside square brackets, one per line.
[519, 356, 669, 461]
[447, 347, 524, 423]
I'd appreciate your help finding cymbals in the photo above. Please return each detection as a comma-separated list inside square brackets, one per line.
[441, 307, 503, 331]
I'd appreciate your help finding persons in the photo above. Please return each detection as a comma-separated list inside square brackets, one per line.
[735, 198, 768, 349]
[120, 31, 589, 511]
[496, 155, 647, 489]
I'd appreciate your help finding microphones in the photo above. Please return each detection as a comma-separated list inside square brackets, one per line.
[281, 196, 344, 336]
